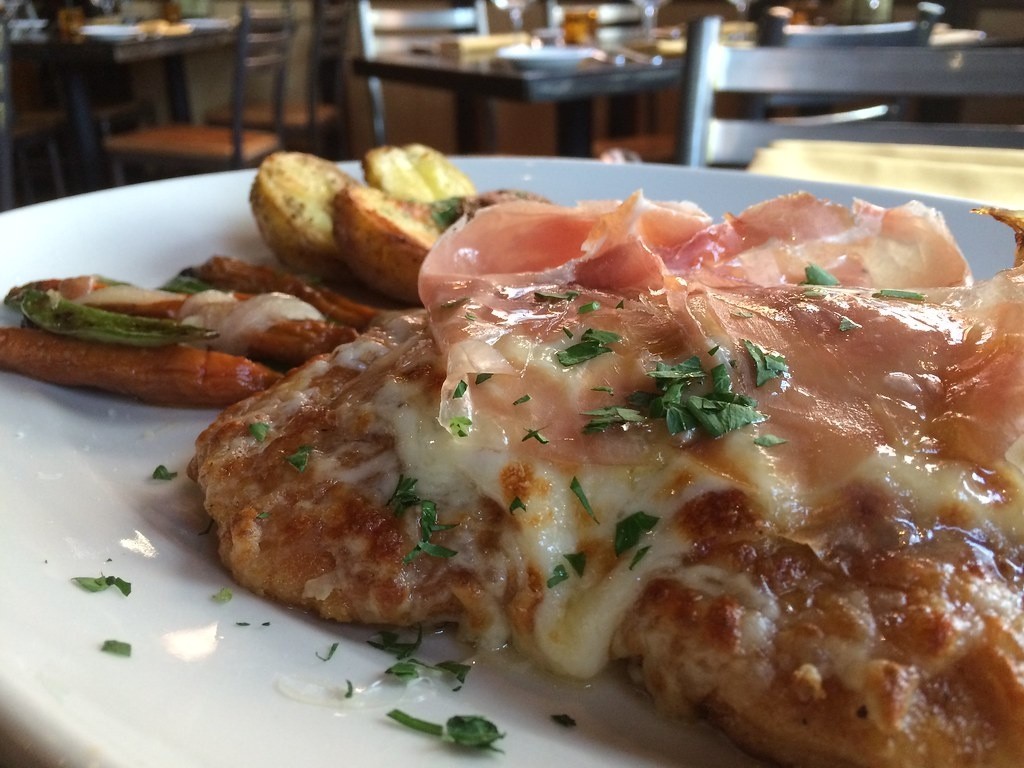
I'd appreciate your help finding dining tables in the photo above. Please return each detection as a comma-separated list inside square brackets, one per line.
[69, 13, 309, 126]
[350, 20, 985, 158]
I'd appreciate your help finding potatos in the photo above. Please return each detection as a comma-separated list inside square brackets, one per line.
[248, 143, 475, 308]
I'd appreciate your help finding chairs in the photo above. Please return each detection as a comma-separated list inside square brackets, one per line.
[544, 1, 660, 156]
[682, 14, 1024, 210]
[739, 1, 947, 125]
[203, 0, 357, 160]
[0, 0, 149, 210]
[357, 0, 492, 160]
[104, 0, 296, 187]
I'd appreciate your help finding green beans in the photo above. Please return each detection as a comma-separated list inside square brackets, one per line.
[12, 287, 221, 347]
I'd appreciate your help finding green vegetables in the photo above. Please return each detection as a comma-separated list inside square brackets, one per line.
[69, 263, 924, 755]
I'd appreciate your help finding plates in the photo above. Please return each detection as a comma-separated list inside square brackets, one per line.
[495, 46, 594, 67]
[79, 24, 144, 41]
[0, 155, 1024, 768]
[182, 18, 230, 30]
[8, 18, 48, 31]
